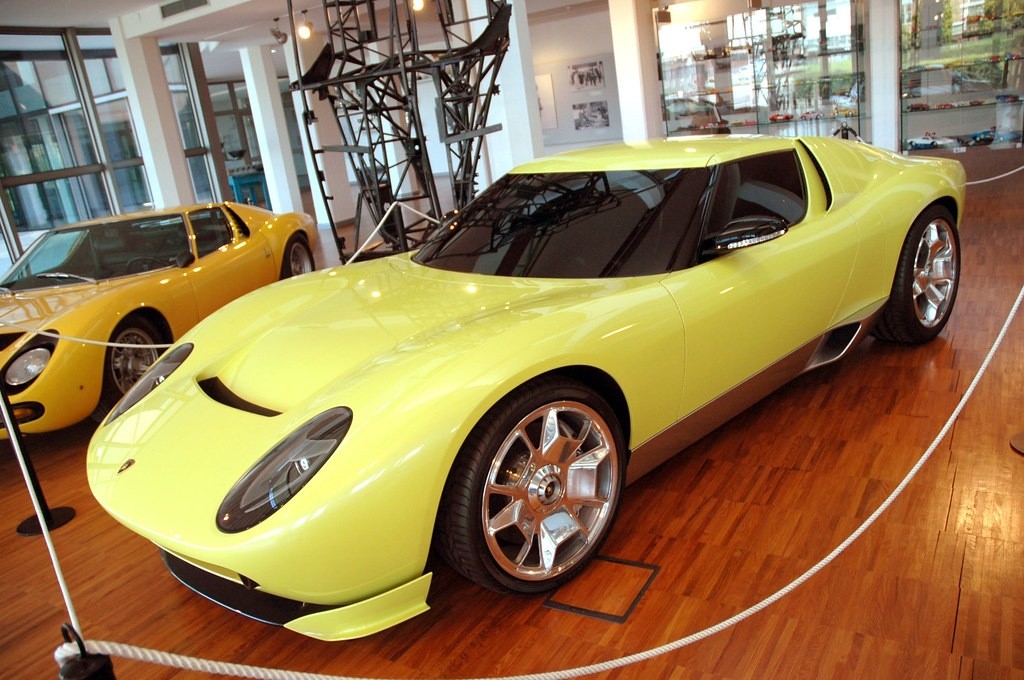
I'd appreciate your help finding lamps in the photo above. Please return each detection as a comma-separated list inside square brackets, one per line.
[270, 19, 287, 44]
[298, 11, 312, 40]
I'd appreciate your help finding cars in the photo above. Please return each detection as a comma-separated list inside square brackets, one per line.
[799, 110, 824, 121]
[699, 120, 731, 134]
[901, 14, 1024, 150]
[691, 74, 865, 96]
[829, 107, 855, 119]
[768, 113, 794, 123]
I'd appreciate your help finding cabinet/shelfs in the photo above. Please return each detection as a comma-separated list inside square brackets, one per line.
[657, 0, 873, 146]
[899, 0, 1024, 156]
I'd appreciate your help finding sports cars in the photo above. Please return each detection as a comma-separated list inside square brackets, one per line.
[1, 201, 320, 443]
[87, 134, 968, 645]
